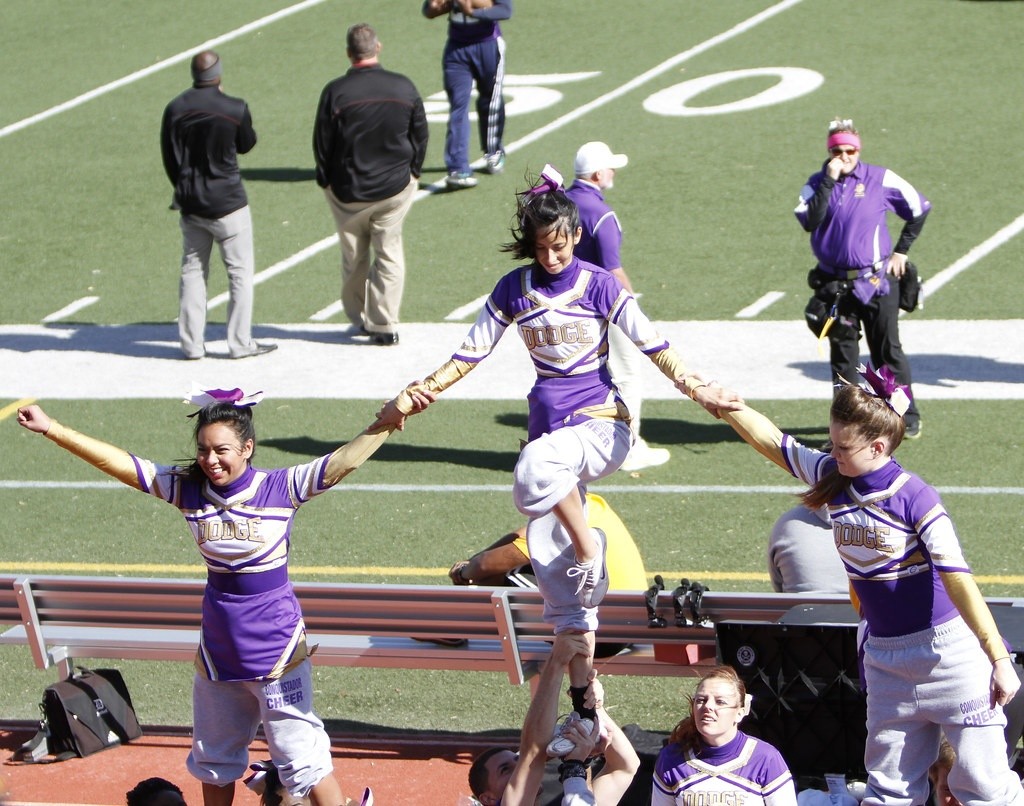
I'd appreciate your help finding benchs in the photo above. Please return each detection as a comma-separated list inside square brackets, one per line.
[2, 572, 1024, 763]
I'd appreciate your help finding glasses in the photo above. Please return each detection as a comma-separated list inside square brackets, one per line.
[829, 148, 857, 157]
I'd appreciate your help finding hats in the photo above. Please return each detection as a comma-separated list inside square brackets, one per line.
[574, 141, 628, 175]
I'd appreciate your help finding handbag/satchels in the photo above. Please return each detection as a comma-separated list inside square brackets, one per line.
[900, 263, 920, 311]
[23, 665, 142, 764]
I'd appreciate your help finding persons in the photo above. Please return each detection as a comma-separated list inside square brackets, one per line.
[368, 158, 746, 756]
[125, 777, 187, 806]
[651, 664, 797, 806]
[468, 669, 640, 806]
[422, 0, 512, 189]
[242, 759, 314, 806]
[766, 440, 850, 594]
[674, 357, 1023, 806]
[410, 493, 650, 658]
[19, 380, 436, 806]
[566, 140, 671, 472]
[312, 22, 428, 346]
[161, 51, 277, 360]
[793, 117, 932, 451]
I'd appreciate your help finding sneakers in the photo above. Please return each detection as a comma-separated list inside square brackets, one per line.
[567, 527, 609, 608]
[546, 711, 601, 756]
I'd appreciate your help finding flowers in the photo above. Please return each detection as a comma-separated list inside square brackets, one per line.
[182, 390, 262, 418]
[524, 162, 564, 198]
[834, 363, 913, 417]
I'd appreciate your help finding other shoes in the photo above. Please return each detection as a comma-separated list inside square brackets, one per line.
[621, 446, 672, 471]
[358, 322, 372, 335]
[483, 147, 507, 173]
[902, 419, 923, 440]
[820, 438, 834, 453]
[411, 634, 470, 648]
[232, 342, 278, 356]
[447, 170, 478, 187]
[369, 331, 398, 346]
